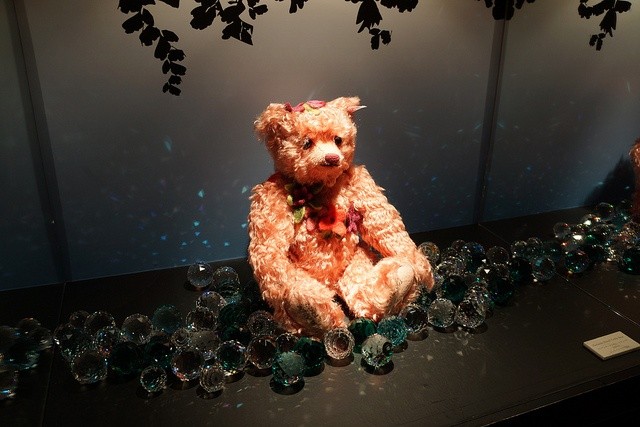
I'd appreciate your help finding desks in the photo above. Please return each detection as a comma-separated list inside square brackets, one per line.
[33, 222, 640, 425]
[481, 201, 640, 329]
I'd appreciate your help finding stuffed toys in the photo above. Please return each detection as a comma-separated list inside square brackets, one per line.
[247, 95, 436, 341]
